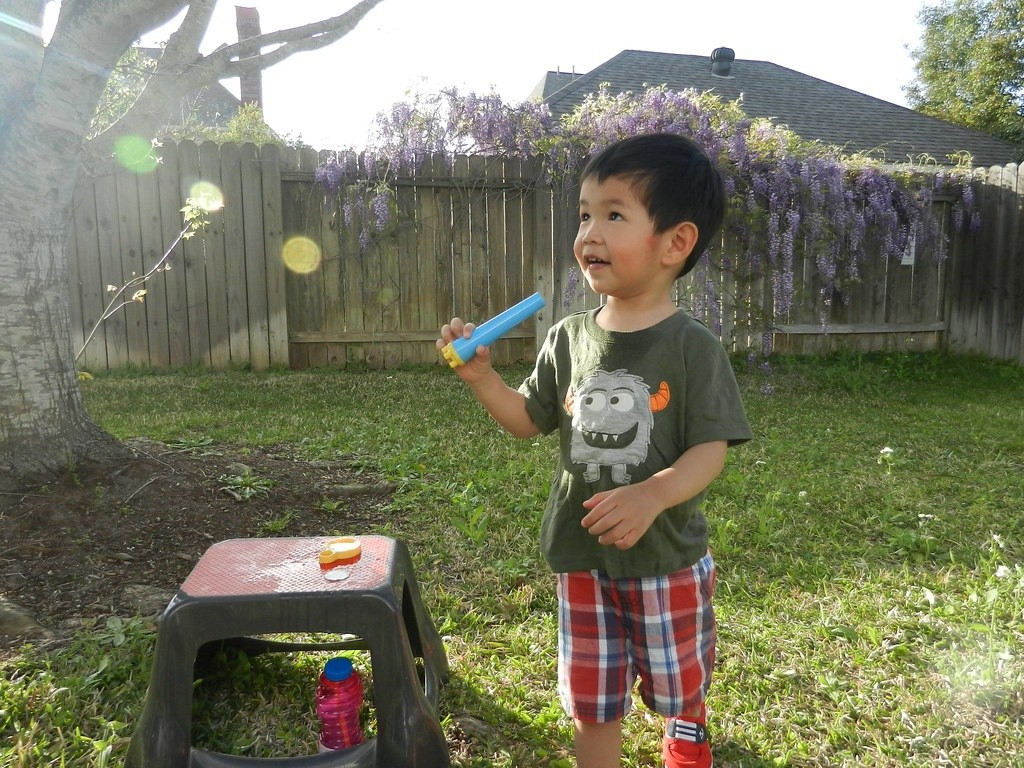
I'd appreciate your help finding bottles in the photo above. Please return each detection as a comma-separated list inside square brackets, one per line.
[315, 657, 367, 753]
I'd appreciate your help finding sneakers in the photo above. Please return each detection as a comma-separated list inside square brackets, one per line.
[663, 703, 713, 768]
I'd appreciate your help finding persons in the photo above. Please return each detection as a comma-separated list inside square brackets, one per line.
[436, 133, 753, 768]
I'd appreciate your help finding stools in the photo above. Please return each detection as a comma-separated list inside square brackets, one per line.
[128, 534, 454, 768]
